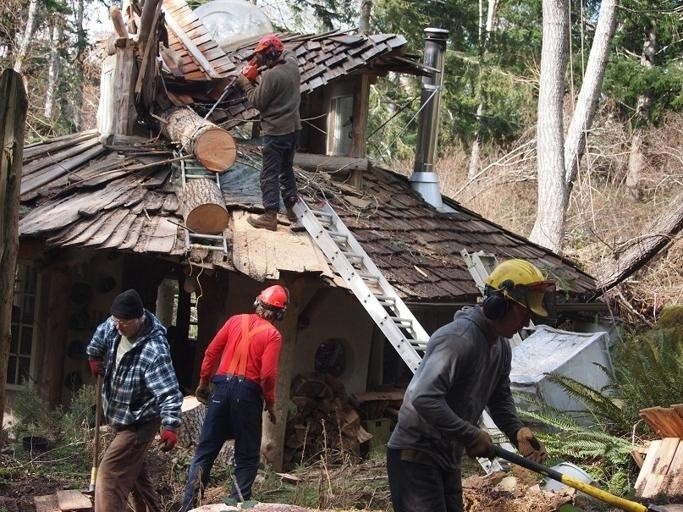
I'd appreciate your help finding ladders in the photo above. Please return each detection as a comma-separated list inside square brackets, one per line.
[460, 248, 537, 346]
[180, 152, 227, 253]
[291, 198, 530, 472]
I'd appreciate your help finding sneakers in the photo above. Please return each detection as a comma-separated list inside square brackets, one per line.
[246, 209, 277, 231]
[285, 208, 297, 221]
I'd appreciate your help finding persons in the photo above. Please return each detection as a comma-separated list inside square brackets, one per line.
[381, 256, 549, 512]
[176, 283, 290, 511]
[82, 288, 185, 511]
[223, 33, 305, 232]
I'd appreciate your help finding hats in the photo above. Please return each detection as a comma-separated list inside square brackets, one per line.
[110, 289, 144, 320]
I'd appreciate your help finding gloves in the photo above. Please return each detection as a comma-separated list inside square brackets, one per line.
[236, 75, 251, 90]
[157, 424, 178, 452]
[513, 426, 548, 465]
[194, 382, 210, 406]
[264, 405, 276, 424]
[466, 430, 495, 461]
[88, 358, 105, 379]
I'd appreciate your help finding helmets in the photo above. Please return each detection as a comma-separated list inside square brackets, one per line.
[485, 258, 550, 317]
[253, 35, 283, 67]
[256, 284, 290, 314]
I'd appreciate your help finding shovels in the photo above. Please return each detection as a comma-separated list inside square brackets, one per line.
[496, 438, 683, 512]
